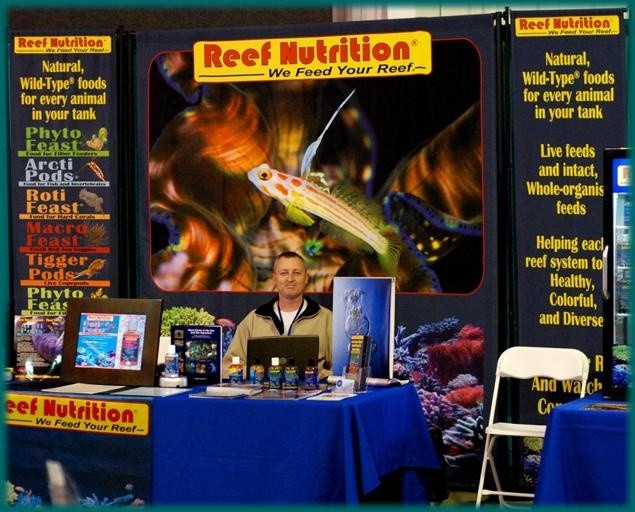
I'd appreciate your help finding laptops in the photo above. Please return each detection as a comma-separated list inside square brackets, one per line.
[246, 335, 319, 387]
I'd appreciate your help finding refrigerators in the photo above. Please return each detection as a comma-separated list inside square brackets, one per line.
[601, 146, 635, 402]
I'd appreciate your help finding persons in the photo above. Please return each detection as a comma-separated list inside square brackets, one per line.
[223, 253, 332, 377]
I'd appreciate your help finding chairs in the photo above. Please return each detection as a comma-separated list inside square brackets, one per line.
[473, 344, 594, 510]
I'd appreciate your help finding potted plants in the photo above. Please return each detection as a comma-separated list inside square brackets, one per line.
[150, 303, 221, 370]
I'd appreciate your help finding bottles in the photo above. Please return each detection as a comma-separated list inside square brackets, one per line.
[303, 358, 319, 390]
[120, 317, 140, 366]
[249, 357, 265, 385]
[284, 358, 297, 390]
[229, 356, 243, 385]
[267, 357, 283, 389]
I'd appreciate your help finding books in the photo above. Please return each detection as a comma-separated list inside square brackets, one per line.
[170, 324, 221, 382]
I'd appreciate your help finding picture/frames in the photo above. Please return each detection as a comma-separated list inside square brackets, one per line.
[55, 294, 164, 390]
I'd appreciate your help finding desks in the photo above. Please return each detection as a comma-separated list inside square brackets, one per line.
[528, 387, 632, 507]
[2, 374, 446, 512]
